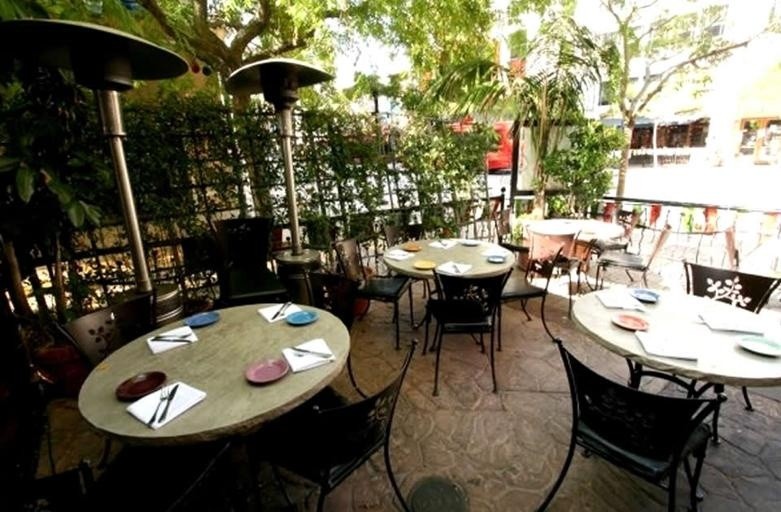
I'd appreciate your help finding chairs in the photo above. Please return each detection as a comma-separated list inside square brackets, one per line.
[239, 339, 417, 511]
[421, 267, 514, 396]
[680, 256, 781, 412]
[210, 217, 288, 310]
[593, 224, 671, 287]
[3, 343, 82, 511]
[529, 229, 581, 294]
[333, 236, 415, 350]
[282, 272, 371, 401]
[521, 199, 779, 267]
[540, 339, 727, 511]
[491, 205, 528, 258]
[497, 236, 566, 351]
[48, 289, 158, 370]
[75, 439, 233, 509]
[385, 237, 516, 356]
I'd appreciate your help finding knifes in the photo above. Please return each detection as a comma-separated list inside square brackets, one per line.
[271, 300, 287, 320]
[454, 265, 462, 274]
[388, 252, 407, 257]
[158, 385, 179, 422]
[439, 238, 447, 247]
[150, 338, 192, 345]
[287, 344, 332, 359]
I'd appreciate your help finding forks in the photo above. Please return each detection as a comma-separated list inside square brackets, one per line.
[153, 333, 192, 338]
[278, 303, 293, 316]
[145, 386, 167, 427]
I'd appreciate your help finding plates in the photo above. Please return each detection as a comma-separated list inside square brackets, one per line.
[461, 239, 479, 247]
[184, 310, 219, 328]
[115, 370, 167, 403]
[401, 243, 423, 252]
[413, 260, 436, 270]
[612, 288, 659, 333]
[737, 334, 781, 359]
[241, 359, 289, 384]
[284, 310, 318, 326]
[487, 255, 505, 264]
